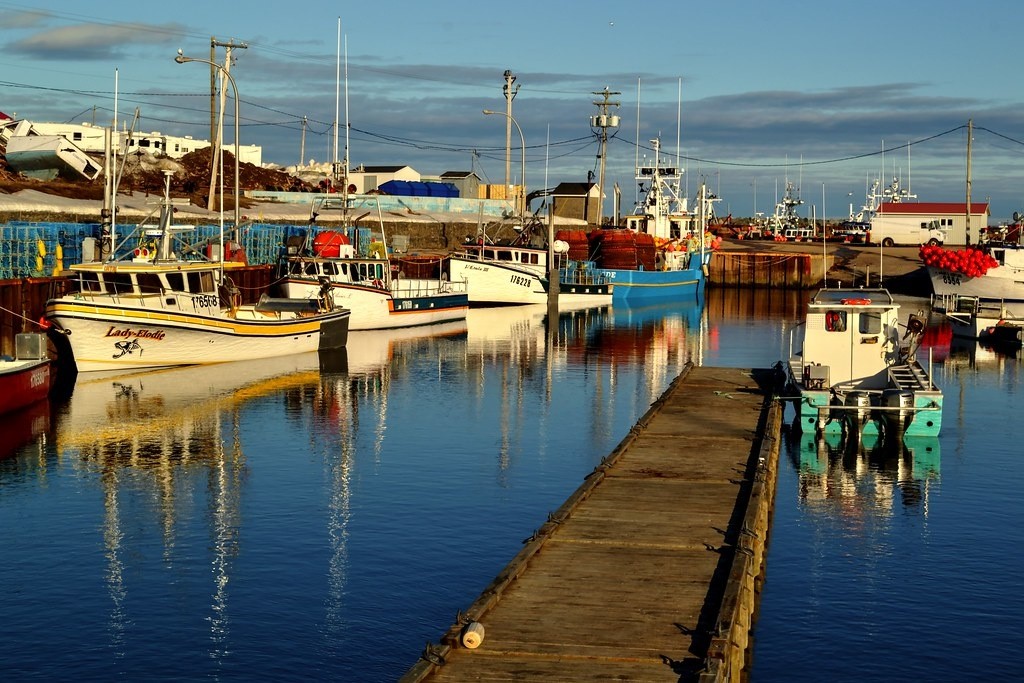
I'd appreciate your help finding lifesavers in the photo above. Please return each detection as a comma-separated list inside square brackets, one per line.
[841, 298, 871, 305]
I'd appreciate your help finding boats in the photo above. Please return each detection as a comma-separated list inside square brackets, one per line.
[435, 244, 712, 304]
[0, 356, 50, 414]
[797, 433, 941, 517]
[787, 289, 945, 437]
[457, 299, 709, 407]
[54, 352, 315, 456]
[945, 298, 1024, 365]
[949, 335, 1024, 381]
[921, 239, 1024, 300]
[46, 169, 351, 372]
[280, 257, 469, 331]
[0, 397, 51, 461]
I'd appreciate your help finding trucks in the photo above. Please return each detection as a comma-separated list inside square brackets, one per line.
[870, 217, 945, 248]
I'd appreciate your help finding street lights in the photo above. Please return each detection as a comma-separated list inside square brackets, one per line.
[174, 57, 242, 243]
[482, 109, 525, 243]
